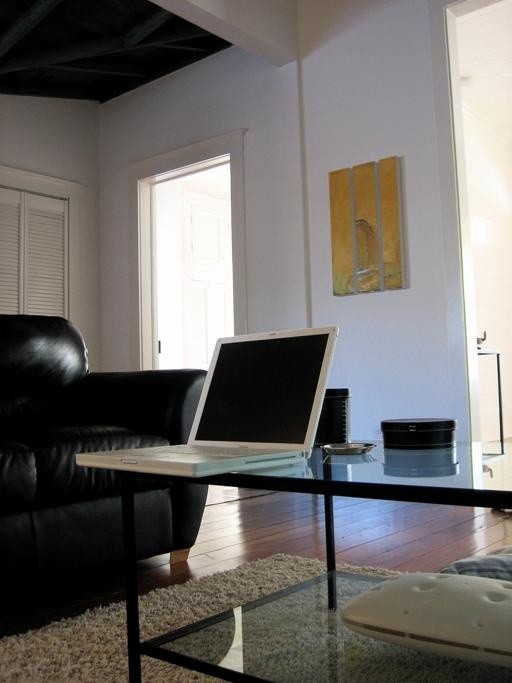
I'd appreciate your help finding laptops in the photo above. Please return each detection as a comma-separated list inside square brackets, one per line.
[74, 326, 339, 480]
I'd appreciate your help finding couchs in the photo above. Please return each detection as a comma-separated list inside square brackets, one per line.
[1, 313, 210, 588]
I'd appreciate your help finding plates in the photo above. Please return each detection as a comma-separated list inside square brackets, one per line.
[322, 442, 376, 454]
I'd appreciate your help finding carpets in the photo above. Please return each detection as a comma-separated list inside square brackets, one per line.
[0, 551, 510, 682]
